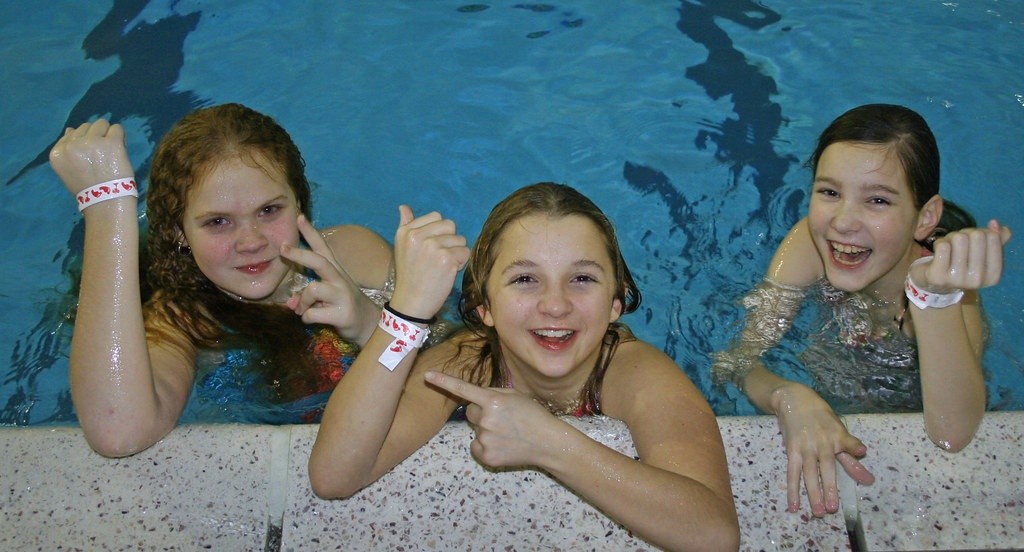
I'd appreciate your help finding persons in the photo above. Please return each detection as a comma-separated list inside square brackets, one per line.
[742, 103, 1011, 517]
[308, 180, 740, 552]
[49, 102, 396, 458]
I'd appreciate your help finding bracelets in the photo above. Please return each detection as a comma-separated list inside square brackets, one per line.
[76, 176, 139, 212]
[904, 255, 964, 309]
[377, 300, 438, 371]
